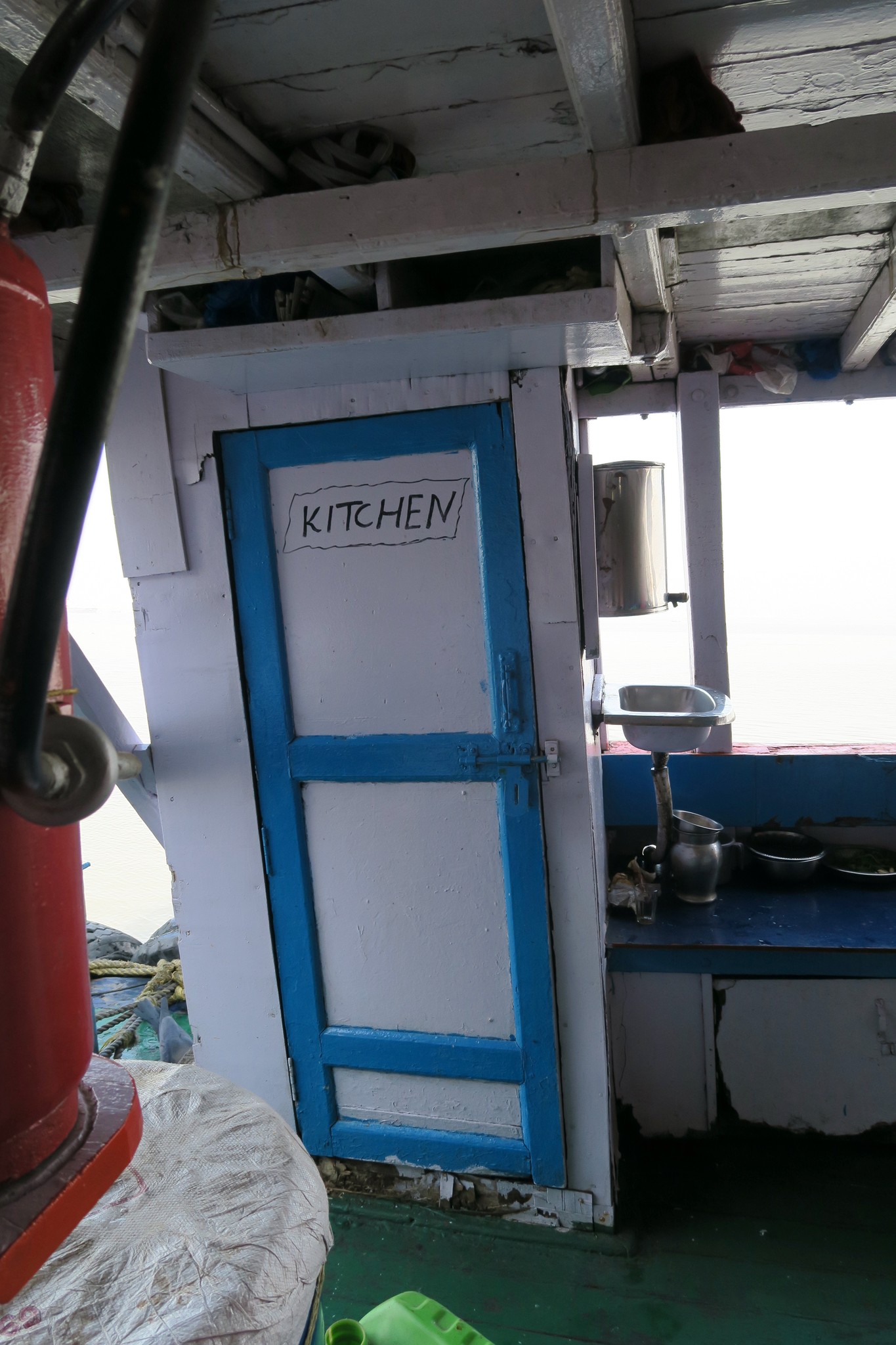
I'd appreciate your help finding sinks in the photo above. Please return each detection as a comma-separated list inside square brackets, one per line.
[601, 680, 740, 755]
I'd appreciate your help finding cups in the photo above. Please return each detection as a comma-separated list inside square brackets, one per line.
[634, 884, 658, 925]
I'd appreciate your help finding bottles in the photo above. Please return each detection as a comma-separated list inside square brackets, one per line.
[669, 826, 724, 903]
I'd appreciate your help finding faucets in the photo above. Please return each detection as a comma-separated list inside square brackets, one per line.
[667, 592, 690, 609]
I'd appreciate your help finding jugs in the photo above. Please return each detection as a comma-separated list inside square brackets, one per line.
[719, 835, 745, 882]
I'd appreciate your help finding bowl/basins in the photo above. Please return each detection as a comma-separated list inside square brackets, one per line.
[747, 831, 826, 880]
[672, 809, 724, 834]
[822, 848, 896, 883]
